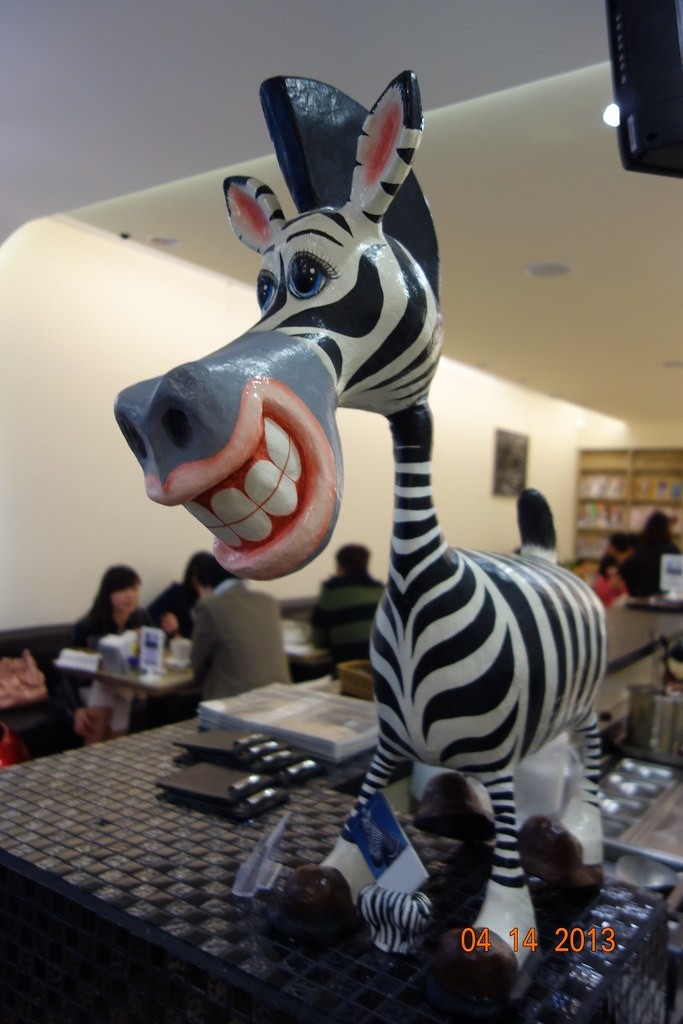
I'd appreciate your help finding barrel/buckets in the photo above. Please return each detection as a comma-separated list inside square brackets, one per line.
[627, 684, 683, 756]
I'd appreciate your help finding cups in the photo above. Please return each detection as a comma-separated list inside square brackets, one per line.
[170, 640, 191, 660]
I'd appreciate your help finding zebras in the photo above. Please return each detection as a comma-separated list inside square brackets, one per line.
[112, 69, 613, 954]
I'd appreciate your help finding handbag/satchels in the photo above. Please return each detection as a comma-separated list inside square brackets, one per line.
[0, 650, 49, 710]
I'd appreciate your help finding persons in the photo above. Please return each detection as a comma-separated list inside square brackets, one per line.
[62, 552, 294, 746]
[312, 548, 385, 673]
[592, 512, 681, 609]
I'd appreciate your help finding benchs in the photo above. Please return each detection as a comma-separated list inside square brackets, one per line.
[0, 623, 69, 753]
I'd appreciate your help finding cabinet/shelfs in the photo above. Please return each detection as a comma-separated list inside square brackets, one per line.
[572, 445, 683, 564]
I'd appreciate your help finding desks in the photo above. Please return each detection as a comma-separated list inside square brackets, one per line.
[280, 617, 333, 664]
[54, 650, 195, 732]
